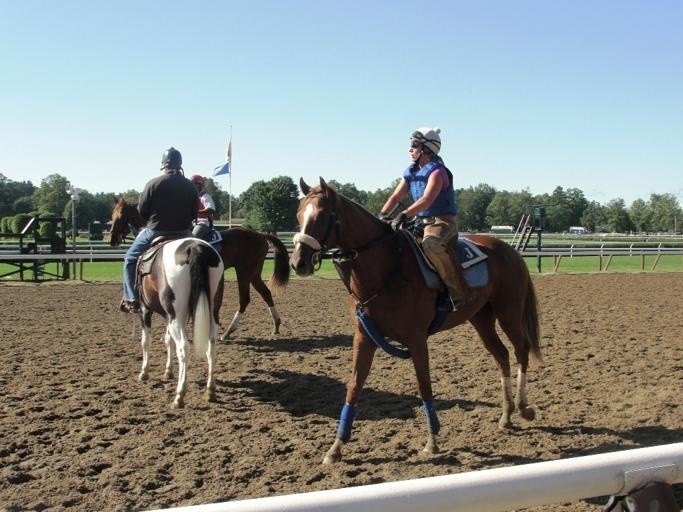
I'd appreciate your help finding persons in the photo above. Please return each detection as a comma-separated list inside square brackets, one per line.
[114, 146, 202, 314]
[191, 174, 217, 242]
[377, 126, 468, 312]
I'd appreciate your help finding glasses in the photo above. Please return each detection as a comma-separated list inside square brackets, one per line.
[411, 140, 423, 148]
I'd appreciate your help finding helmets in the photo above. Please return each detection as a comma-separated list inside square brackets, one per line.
[191, 175, 204, 184]
[410, 127, 441, 154]
[162, 147, 182, 167]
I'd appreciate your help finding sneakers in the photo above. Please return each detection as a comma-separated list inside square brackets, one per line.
[438, 291, 474, 313]
[123, 302, 140, 312]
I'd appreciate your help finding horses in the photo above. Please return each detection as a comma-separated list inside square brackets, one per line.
[285, 174, 544, 467]
[134, 231, 227, 411]
[108, 192, 292, 341]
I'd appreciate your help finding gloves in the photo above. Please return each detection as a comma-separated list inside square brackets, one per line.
[391, 213, 405, 231]
